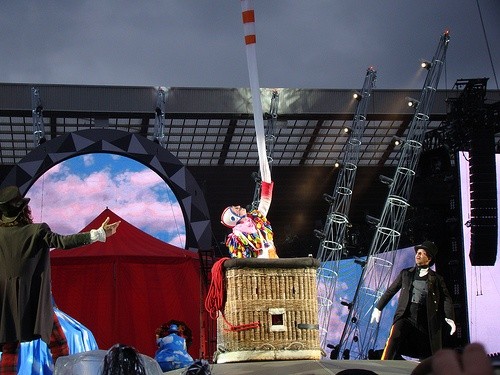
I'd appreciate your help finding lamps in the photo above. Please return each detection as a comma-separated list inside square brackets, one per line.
[38, 136, 47, 145]
[33, 105, 43, 117]
[421, 62, 431, 70]
[407, 100, 417, 109]
[353, 93, 362, 101]
[335, 162, 343, 169]
[344, 127, 352, 134]
[394, 140, 401, 145]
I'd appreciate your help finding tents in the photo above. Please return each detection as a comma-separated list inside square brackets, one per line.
[49, 208, 210, 362]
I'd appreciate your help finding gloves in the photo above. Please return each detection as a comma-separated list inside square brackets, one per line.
[370, 307, 382, 323]
[445, 318, 457, 336]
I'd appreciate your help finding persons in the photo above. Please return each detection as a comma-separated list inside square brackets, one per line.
[335, 241, 492, 375]
[0, 186, 210, 375]
[221, 181, 281, 260]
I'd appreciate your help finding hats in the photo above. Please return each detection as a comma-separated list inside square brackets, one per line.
[0, 185, 31, 224]
[414, 241, 438, 267]
[220, 206, 241, 229]
